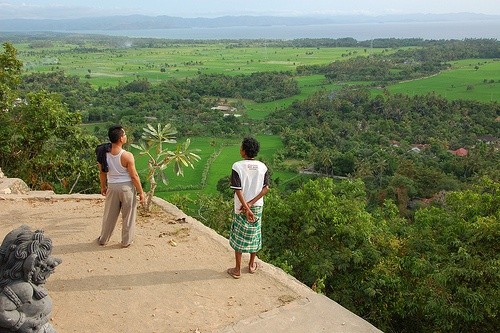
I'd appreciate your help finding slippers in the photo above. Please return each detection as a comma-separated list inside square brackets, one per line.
[249, 262, 260, 274]
[227, 268, 241, 279]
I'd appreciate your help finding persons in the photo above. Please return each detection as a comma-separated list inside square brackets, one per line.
[227, 138, 269, 278]
[95, 125, 144, 248]
